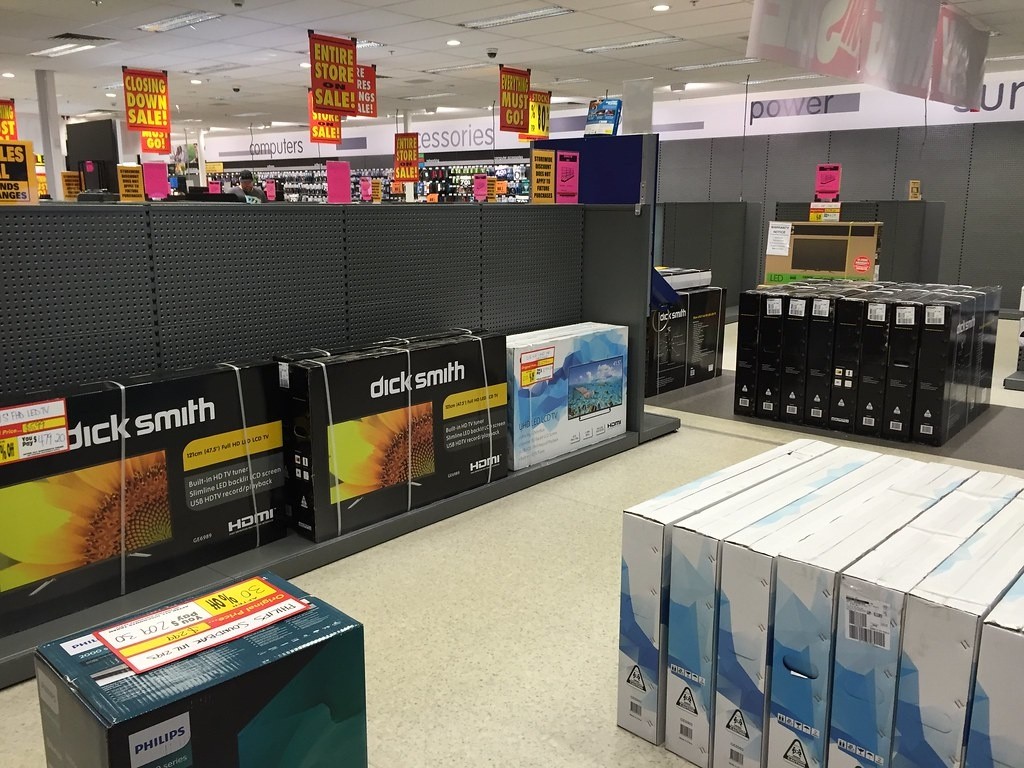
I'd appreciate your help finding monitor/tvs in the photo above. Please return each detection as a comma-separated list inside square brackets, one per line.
[568, 356, 622, 421]
[65, 119, 119, 193]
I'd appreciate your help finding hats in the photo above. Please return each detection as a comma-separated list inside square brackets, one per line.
[239, 169, 253, 181]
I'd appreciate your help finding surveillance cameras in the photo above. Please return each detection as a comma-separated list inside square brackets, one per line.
[486, 48, 498, 59]
[233, 88, 239, 92]
[232, 0, 244, 8]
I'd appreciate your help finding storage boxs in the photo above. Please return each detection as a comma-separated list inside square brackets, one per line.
[0, 267, 1023, 768]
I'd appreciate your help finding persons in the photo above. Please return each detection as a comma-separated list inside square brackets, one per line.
[226, 169, 268, 205]
[174, 145, 185, 163]
[189, 142, 199, 163]
